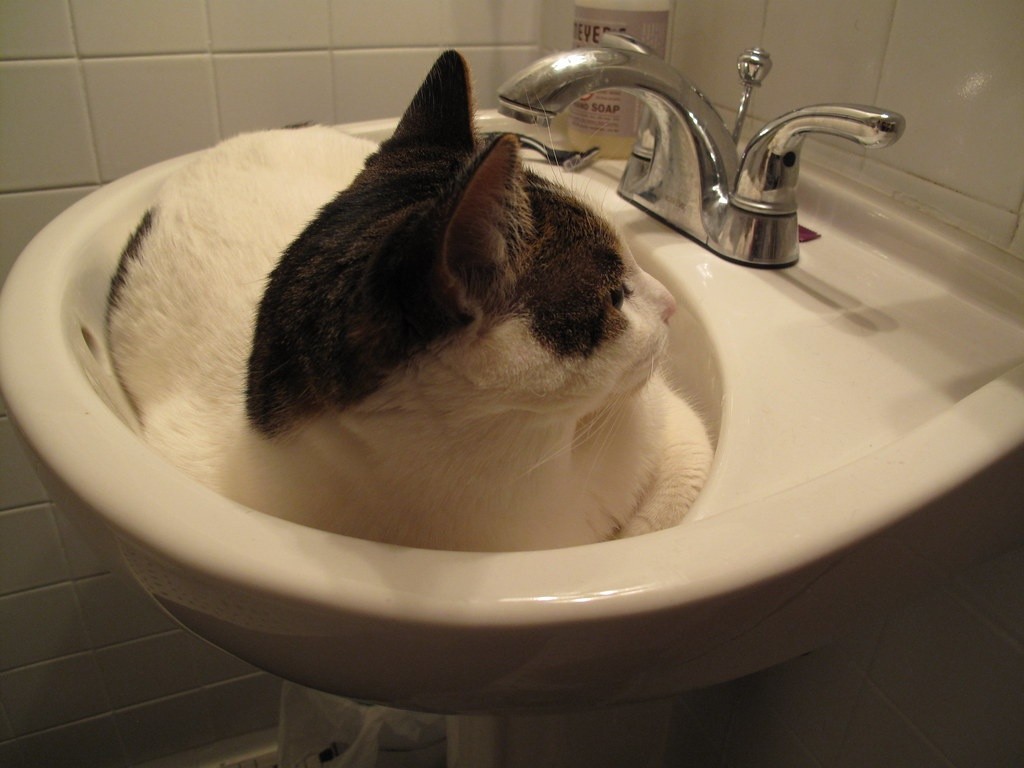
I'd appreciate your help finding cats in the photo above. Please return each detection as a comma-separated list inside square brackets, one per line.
[105, 49, 712, 552]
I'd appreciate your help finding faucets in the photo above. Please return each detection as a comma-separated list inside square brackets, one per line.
[494, 45, 743, 244]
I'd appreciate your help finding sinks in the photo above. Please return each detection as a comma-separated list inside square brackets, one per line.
[2, 105, 1023, 720]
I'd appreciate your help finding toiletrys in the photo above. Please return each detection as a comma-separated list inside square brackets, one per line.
[566, 0, 676, 158]
